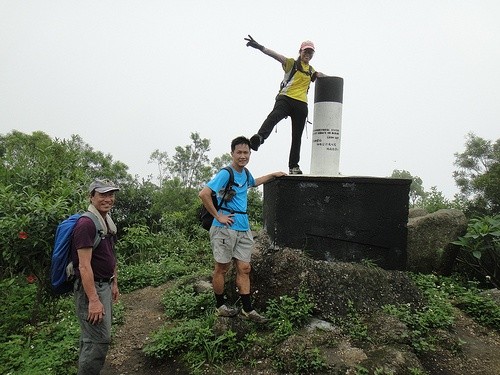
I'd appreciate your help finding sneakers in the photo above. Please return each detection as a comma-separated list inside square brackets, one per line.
[239, 309, 266, 323]
[289, 165, 302, 174]
[249, 134, 265, 151]
[214, 304, 239, 317]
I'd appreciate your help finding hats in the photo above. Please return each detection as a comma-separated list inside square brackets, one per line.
[89, 179, 120, 193]
[300, 43, 315, 52]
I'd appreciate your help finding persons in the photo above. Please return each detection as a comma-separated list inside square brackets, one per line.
[52, 178, 120, 375]
[198, 135, 288, 324]
[243, 33, 325, 176]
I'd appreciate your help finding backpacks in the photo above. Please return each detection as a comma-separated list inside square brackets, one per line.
[198, 166, 249, 231]
[50, 213, 104, 288]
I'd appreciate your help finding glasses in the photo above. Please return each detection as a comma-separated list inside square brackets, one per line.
[302, 49, 314, 54]
